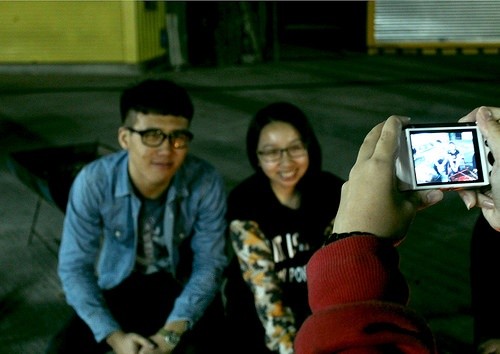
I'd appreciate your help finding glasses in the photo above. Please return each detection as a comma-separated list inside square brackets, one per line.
[254, 139, 310, 162]
[123, 126, 193, 150]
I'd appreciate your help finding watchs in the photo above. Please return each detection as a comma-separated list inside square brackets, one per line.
[157, 327, 179, 347]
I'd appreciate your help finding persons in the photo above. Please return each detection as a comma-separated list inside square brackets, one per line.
[228, 102, 349, 353]
[295, 107, 500, 354]
[58, 79, 227, 354]
[431, 138, 461, 177]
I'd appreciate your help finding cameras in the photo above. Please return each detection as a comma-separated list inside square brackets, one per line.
[396, 122, 495, 192]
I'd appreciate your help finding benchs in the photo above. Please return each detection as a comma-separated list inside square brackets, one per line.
[11, 142, 120, 261]
